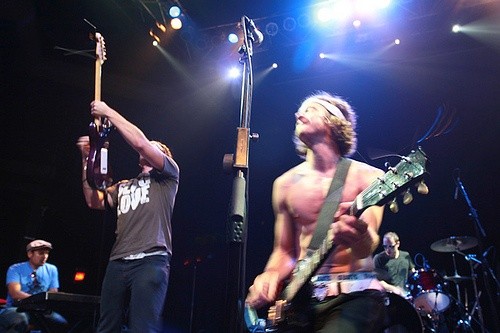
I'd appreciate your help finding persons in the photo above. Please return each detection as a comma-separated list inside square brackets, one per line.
[78, 102, 178, 333]
[0, 240, 67, 333]
[373, 232, 423, 297]
[245, 95, 386, 333]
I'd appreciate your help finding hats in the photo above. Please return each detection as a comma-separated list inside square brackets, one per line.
[25, 240, 53, 252]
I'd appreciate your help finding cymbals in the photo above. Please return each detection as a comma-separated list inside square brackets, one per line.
[431, 235, 481, 252]
[443, 274, 474, 285]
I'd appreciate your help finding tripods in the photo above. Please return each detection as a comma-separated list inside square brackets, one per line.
[438, 250, 498, 333]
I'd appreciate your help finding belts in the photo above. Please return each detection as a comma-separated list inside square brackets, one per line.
[123, 249, 168, 260]
[310, 278, 384, 302]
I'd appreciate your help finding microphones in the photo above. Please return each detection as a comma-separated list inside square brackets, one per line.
[453, 177, 459, 199]
[244, 16, 264, 45]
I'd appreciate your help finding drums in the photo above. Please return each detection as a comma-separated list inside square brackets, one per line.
[373, 290, 425, 333]
[408, 268, 452, 315]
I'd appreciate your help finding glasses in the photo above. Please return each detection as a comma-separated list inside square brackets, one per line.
[383, 243, 396, 249]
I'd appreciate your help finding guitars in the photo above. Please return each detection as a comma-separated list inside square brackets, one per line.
[85, 31, 114, 191]
[244, 145, 434, 333]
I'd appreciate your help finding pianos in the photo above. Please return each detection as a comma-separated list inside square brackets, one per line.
[17, 291, 102, 333]
